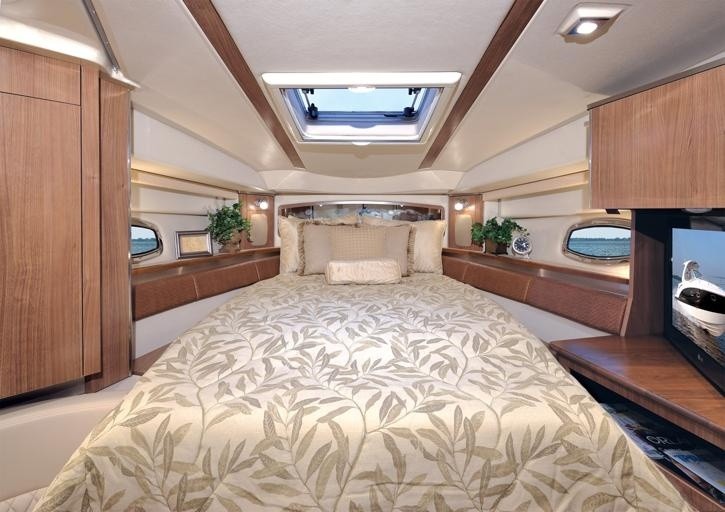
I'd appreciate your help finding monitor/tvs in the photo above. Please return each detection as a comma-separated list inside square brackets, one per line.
[661, 212, 724, 402]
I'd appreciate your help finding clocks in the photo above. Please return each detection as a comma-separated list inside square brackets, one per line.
[513, 237, 533, 254]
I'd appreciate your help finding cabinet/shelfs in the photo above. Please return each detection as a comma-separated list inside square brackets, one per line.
[0, 38, 102, 401]
[549, 334, 725, 512]
[587, 58, 725, 208]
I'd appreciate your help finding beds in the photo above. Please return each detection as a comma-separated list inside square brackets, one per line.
[30, 215, 696, 511]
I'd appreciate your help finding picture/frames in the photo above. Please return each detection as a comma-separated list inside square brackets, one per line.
[175, 231, 213, 259]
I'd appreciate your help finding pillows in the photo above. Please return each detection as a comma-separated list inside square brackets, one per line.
[276, 215, 448, 285]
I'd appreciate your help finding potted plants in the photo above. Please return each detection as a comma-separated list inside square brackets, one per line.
[471, 215, 530, 254]
[203, 199, 252, 253]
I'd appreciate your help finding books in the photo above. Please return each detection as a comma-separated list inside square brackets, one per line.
[599, 398, 725, 507]
